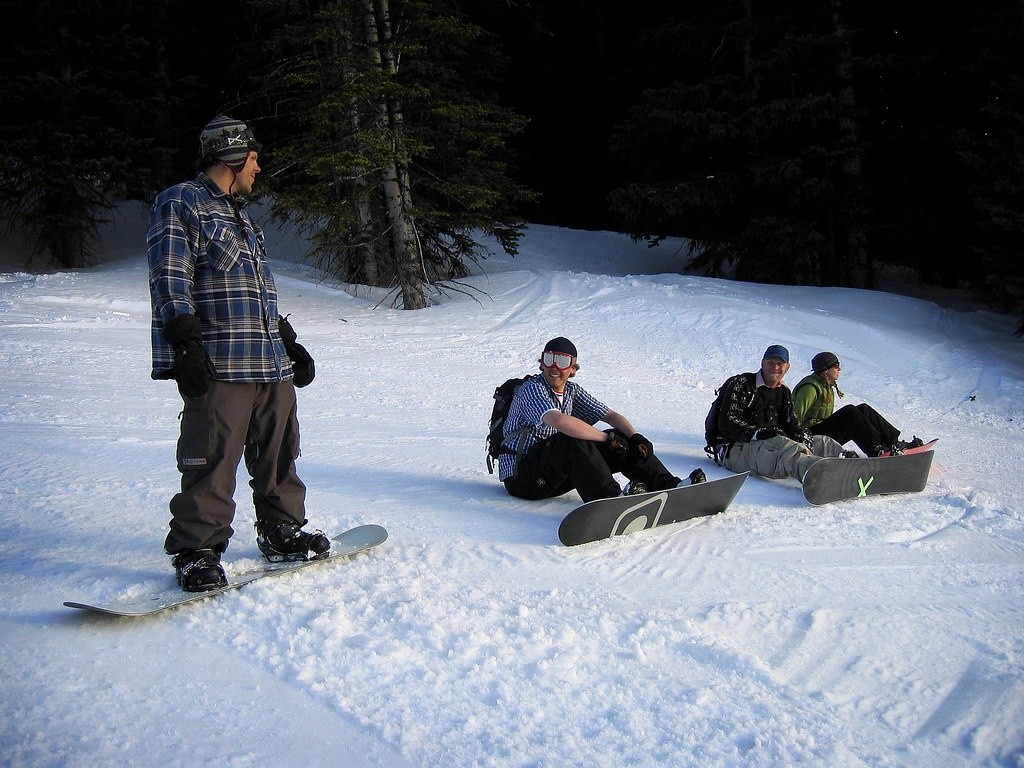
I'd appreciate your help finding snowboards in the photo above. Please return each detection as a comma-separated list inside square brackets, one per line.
[62, 524, 390, 619]
[557, 469, 753, 547]
[879, 436, 941, 457]
[801, 450, 935, 506]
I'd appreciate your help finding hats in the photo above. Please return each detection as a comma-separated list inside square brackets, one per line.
[811, 352, 839, 372]
[543, 336, 578, 358]
[199, 114, 262, 208]
[763, 345, 789, 364]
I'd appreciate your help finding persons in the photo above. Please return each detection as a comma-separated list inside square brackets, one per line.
[498, 336, 708, 505]
[792, 351, 925, 458]
[718, 345, 860, 483]
[147, 114, 329, 593]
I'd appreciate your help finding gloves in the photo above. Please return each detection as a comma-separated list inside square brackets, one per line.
[756, 428, 788, 440]
[160, 314, 218, 399]
[606, 431, 630, 462]
[797, 428, 815, 452]
[276, 314, 316, 388]
[629, 433, 653, 462]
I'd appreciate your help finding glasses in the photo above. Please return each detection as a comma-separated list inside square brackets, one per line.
[541, 352, 572, 371]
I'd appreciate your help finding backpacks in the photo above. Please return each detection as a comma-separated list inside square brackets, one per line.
[704, 372, 757, 448]
[488, 375, 553, 459]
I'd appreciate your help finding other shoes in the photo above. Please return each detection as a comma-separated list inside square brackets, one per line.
[867, 442, 903, 459]
[894, 435, 924, 452]
[842, 449, 860, 459]
[615, 479, 644, 498]
[676, 468, 707, 488]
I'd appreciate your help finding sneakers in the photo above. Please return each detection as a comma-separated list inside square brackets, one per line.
[256, 521, 331, 563]
[176, 545, 228, 593]
